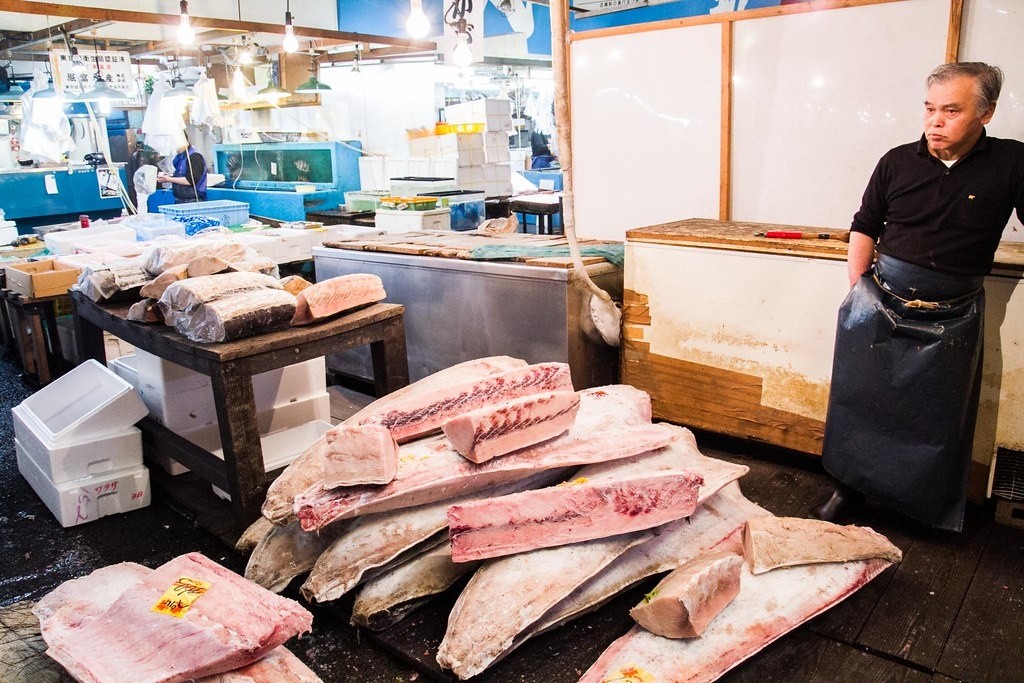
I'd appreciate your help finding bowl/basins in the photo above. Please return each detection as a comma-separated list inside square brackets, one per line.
[18, 233, 39, 243]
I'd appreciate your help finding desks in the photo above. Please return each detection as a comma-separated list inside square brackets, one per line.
[63, 282, 409, 536]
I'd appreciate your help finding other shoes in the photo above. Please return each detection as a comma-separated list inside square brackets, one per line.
[805, 482, 863, 522]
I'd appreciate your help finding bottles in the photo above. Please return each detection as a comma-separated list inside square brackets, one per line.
[121, 208, 128, 217]
[80, 214, 89, 228]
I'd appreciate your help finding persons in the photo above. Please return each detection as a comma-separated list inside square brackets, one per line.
[805, 61, 1024, 522]
[156, 130, 208, 205]
[530, 130, 555, 169]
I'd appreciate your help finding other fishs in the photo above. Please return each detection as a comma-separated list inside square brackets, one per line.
[233, 168, 243, 178]
[459, 203, 480, 222]
[293, 158, 311, 172]
[233, 350, 902, 683]
[298, 173, 311, 181]
[228, 154, 238, 171]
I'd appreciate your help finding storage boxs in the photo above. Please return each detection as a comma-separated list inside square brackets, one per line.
[1, 98, 515, 531]
